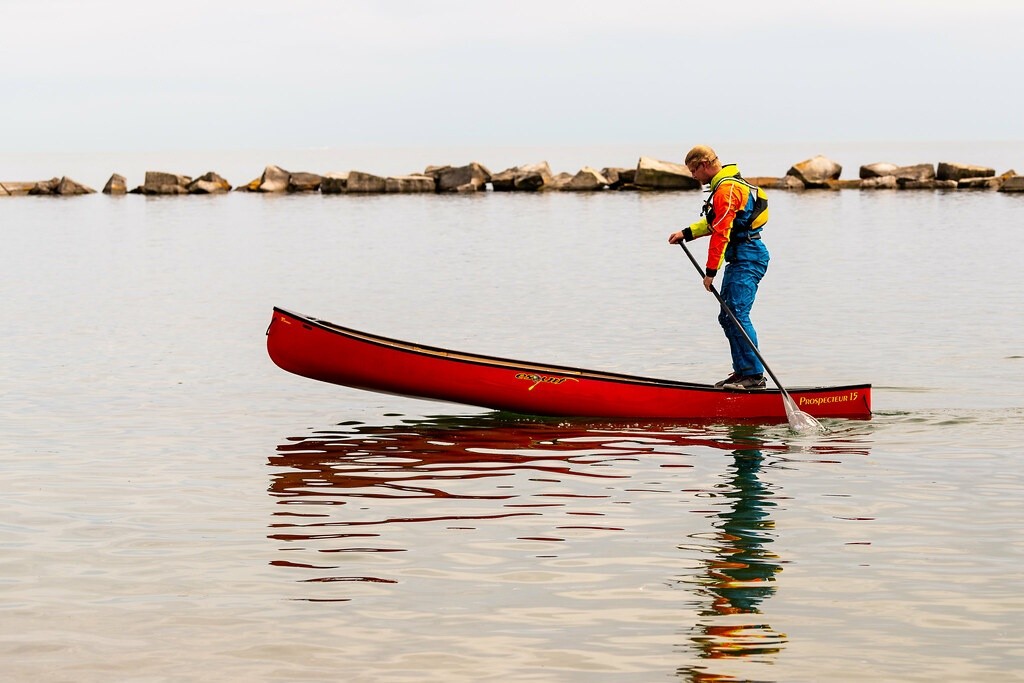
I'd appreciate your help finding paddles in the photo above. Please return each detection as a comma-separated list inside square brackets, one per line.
[670, 237, 826, 430]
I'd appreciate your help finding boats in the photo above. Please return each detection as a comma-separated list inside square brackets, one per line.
[265, 306, 873, 421]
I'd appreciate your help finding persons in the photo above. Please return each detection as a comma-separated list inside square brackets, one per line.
[668, 145, 771, 390]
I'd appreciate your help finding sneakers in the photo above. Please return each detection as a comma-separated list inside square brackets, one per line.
[724, 376, 766, 389]
[714, 373, 741, 388]
